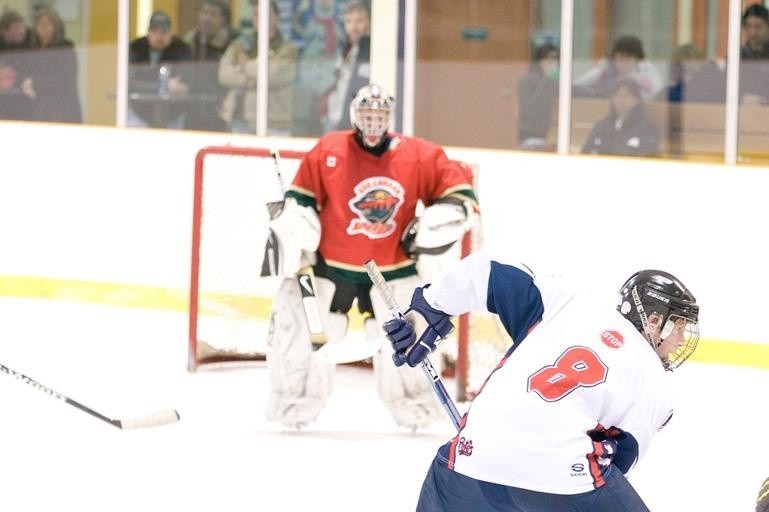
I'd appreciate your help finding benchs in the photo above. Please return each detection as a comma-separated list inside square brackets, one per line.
[542, 93, 769, 157]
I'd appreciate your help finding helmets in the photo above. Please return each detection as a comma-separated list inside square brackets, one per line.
[617, 269, 700, 371]
[349, 84, 393, 147]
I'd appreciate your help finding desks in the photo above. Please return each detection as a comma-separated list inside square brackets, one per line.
[128, 91, 224, 131]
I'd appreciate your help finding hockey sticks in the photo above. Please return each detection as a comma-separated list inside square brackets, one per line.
[268, 147, 327, 348]
[0, 362, 182, 428]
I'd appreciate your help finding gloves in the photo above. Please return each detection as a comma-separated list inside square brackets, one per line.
[383, 284, 457, 367]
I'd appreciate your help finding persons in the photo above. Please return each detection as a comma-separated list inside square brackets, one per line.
[1, 10, 83, 124]
[518, 3, 769, 156]
[128, 1, 371, 138]
[382, 255, 699, 512]
[269, 86, 480, 428]
